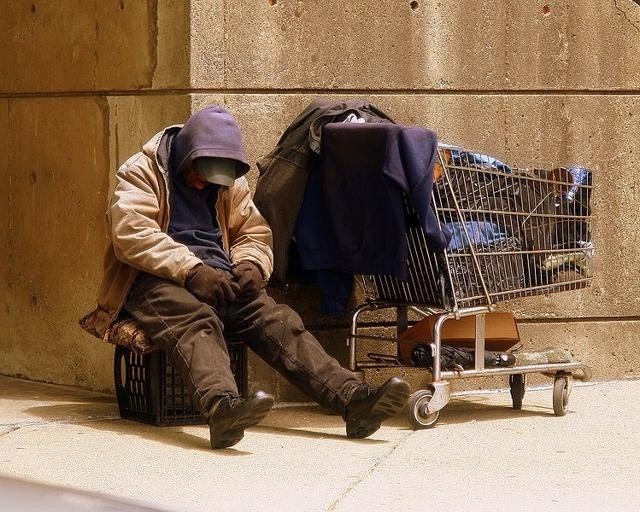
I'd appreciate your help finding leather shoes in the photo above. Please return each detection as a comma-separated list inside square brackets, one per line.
[344, 376, 411, 438]
[210, 390, 274, 449]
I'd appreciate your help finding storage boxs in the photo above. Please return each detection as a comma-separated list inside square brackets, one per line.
[398, 312, 521, 361]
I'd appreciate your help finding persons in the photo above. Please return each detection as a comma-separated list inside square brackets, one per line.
[90, 104, 412, 450]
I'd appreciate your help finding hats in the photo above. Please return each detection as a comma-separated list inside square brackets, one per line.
[195, 156, 237, 187]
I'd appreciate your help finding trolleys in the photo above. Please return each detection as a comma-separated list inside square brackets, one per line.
[312, 119, 600, 428]
